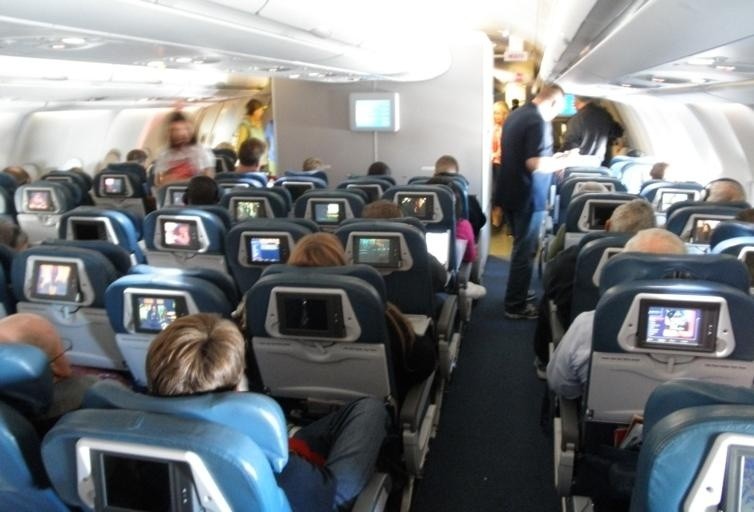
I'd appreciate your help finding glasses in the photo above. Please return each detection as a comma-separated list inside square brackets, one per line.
[48, 334, 73, 367]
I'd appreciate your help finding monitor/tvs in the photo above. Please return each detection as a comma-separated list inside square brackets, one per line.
[662, 193, 688, 212]
[286, 184, 310, 203]
[739, 456, 752, 511]
[237, 202, 260, 221]
[313, 203, 339, 224]
[172, 192, 186, 206]
[250, 238, 280, 262]
[135, 295, 176, 330]
[164, 222, 191, 246]
[358, 238, 391, 264]
[401, 197, 426, 218]
[646, 305, 704, 345]
[76, 223, 98, 240]
[355, 100, 391, 128]
[593, 205, 615, 227]
[99, 454, 169, 511]
[36, 263, 70, 297]
[283, 297, 328, 332]
[28, 191, 48, 210]
[695, 218, 721, 242]
[103, 177, 124, 194]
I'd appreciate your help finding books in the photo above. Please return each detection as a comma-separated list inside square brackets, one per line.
[614, 410, 644, 452]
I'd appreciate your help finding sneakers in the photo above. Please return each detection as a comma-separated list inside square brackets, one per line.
[504, 303, 541, 320]
[525, 289, 537, 301]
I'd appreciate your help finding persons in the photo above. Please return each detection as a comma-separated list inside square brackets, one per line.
[146, 313, 391, 511]
[0, 313, 102, 423]
[175, 224, 190, 246]
[3, 165, 31, 186]
[429, 179, 488, 300]
[0, 212, 31, 252]
[558, 96, 624, 169]
[94, 99, 270, 207]
[146, 304, 159, 329]
[435, 157, 486, 244]
[492, 84, 565, 320]
[533, 161, 754, 402]
[363, 201, 453, 316]
[288, 231, 440, 395]
[37, 264, 68, 298]
